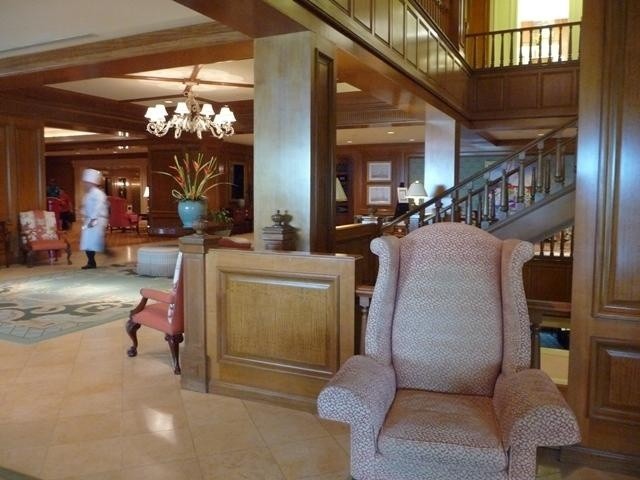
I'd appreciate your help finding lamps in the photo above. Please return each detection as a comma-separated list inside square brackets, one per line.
[143, 186, 149, 212]
[335, 177, 348, 202]
[145, 78, 236, 140]
[404, 180, 427, 206]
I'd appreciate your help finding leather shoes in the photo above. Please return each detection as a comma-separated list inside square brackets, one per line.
[82, 265, 96, 269]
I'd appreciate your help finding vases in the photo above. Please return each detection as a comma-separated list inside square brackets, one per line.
[178, 200, 208, 229]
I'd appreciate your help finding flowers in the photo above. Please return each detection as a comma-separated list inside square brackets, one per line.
[149, 150, 240, 199]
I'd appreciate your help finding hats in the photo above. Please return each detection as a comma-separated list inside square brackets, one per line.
[82, 168, 103, 185]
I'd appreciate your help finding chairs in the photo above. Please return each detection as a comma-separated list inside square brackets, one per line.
[125, 252, 183, 375]
[316, 222, 583, 480]
[0, 192, 140, 269]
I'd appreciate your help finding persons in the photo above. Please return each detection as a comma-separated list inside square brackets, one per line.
[47, 177, 60, 197]
[57, 188, 73, 231]
[97, 184, 117, 257]
[76, 180, 108, 269]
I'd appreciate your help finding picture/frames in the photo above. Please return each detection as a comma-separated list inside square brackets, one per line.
[361, 157, 394, 208]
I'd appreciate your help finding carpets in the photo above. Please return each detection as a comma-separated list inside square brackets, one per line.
[0, 261, 173, 345]
